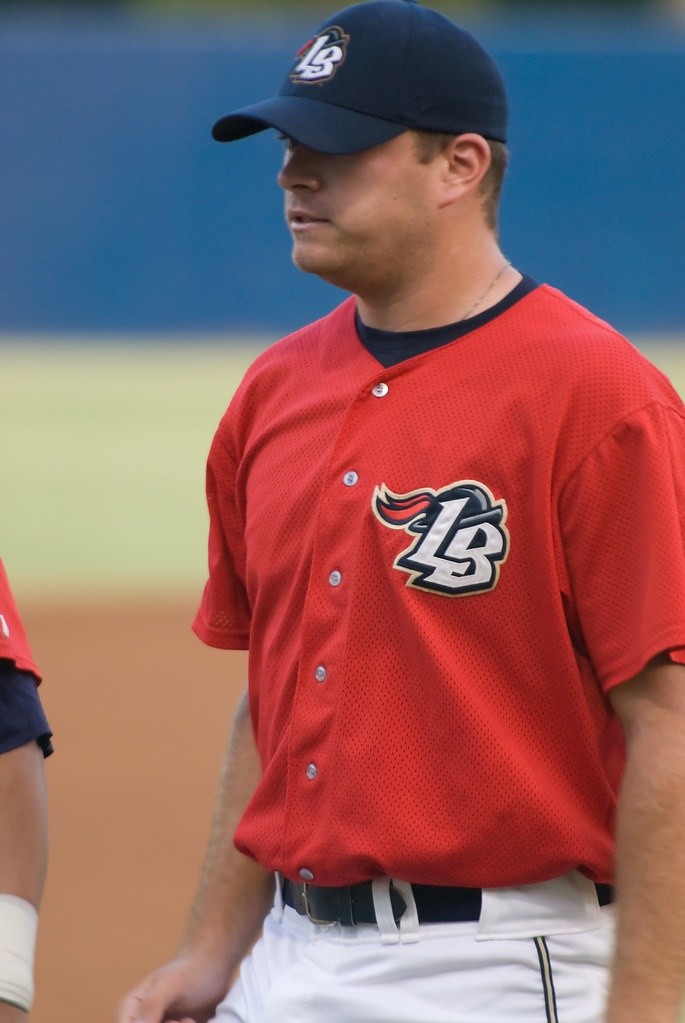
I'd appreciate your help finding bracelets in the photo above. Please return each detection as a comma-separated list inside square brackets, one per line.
[0, 894, 39, 1013]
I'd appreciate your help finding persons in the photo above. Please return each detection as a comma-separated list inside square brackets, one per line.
[113, 0, 685, 1022]
[0, 553, 59, 1023]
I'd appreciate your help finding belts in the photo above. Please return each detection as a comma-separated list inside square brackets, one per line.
[279, 871, 614, 928]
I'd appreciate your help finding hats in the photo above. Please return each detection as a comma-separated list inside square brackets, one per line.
[211, 0, 507, 154]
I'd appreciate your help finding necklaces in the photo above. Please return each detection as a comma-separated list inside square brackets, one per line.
[460, 261, 512, 321]
